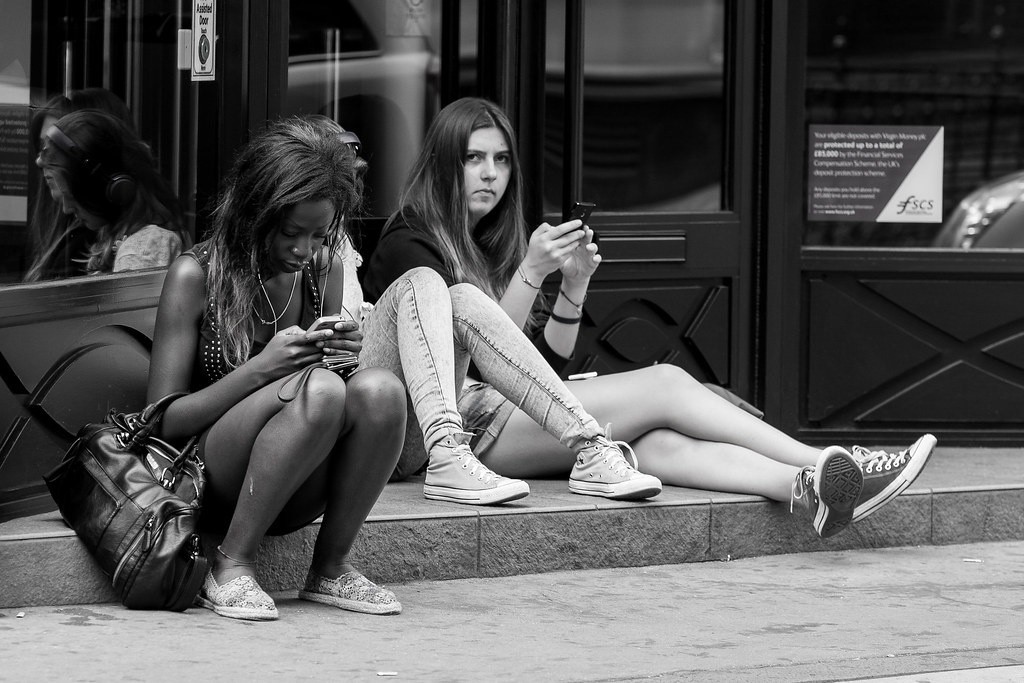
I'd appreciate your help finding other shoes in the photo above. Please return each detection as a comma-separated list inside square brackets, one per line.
[298, 571, 402, 615]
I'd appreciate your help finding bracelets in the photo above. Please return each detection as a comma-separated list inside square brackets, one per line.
[550, 312, 584, 324]
[518, 263, 542, 290]
[558, 285, 587, 314]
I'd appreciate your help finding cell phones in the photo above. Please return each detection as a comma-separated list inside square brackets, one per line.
[305, 316, 346, 333]
[563, 201, 597, 235]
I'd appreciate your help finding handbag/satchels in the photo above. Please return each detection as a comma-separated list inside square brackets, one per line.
[41, 389, 209, 611]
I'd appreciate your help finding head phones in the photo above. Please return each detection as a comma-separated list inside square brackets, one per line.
[44, 123, 138, 212]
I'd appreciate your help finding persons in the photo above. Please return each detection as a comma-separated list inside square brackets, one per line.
[364, 97, 936, 539]
[21, 87, 140, 283]
[144, 114, 412, 620]
[299, 116, 664, 505]
[38, 110, 193, 287]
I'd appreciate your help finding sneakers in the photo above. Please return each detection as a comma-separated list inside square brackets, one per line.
[795, 445, 864, 538]
[195, 567, 279, 621]
[423, 431, 530, 506]
[846, 433, 938, 524]
[567, 436, 663, 500]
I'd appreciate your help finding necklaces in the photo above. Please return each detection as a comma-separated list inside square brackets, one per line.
[248, 260, 301, 325]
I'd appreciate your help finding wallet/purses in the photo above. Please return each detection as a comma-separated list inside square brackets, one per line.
[277, 353, 359, 403]
